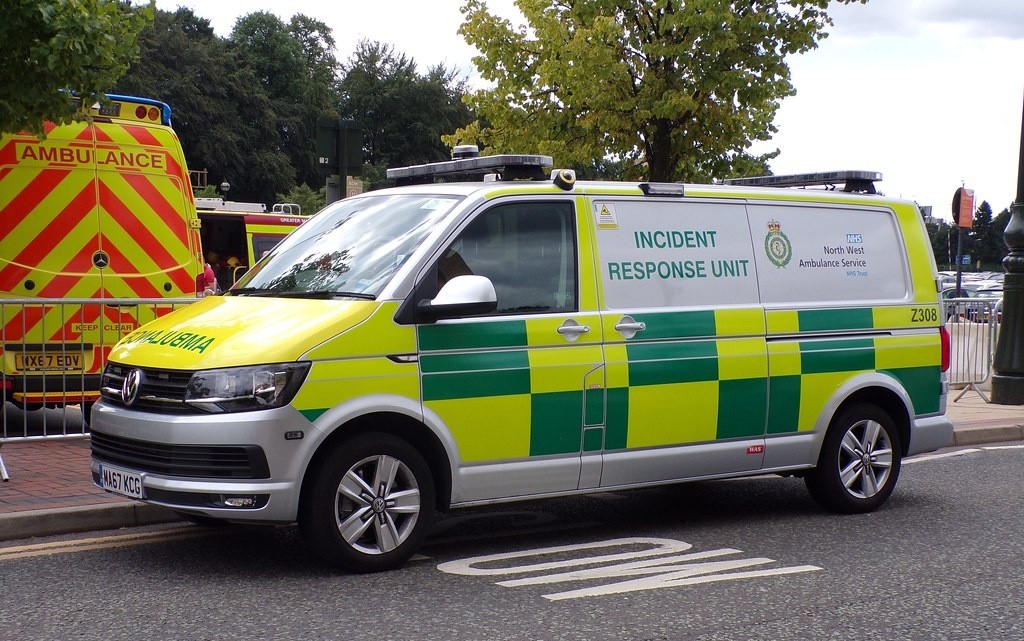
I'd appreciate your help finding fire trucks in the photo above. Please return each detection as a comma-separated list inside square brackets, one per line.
[194, 197, 314, 298]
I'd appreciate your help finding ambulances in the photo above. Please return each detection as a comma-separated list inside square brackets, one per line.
[90, 144, 953, 569]
[0, 86, 216, 427]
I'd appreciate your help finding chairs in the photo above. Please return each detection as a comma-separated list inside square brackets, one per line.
[501, 209, 563, 310]
[463, 215, 494, 277]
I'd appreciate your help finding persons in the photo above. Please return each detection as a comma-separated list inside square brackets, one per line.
[205, 250, 270, 291]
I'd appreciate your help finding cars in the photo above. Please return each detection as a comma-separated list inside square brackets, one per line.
[937, 270, 1004, 324]
